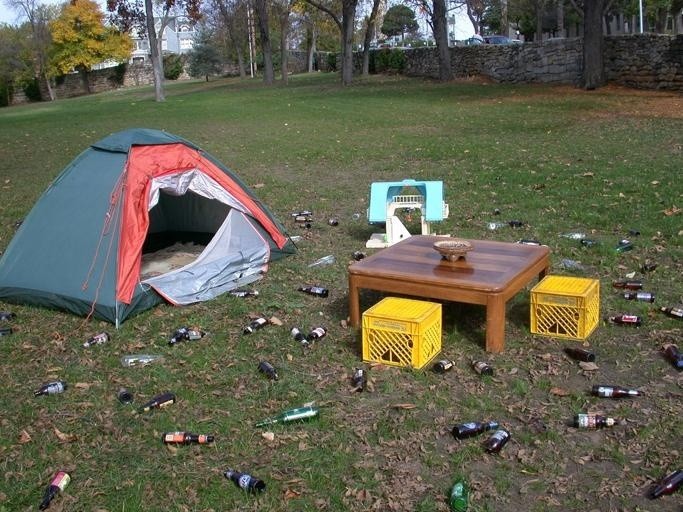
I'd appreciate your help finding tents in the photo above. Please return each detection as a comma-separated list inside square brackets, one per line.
[0, 129, 300, 332]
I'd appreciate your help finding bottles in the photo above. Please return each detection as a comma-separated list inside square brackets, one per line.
[450, 468, 469, 512]
[516, 240, 542, 248]
[493, 208, 502, 216]
[641, 263, 659, 274]
[308, 254, 336, 269]
[619, 240, 631, 246]
[167, 325, 190, 347]
[353, 369, 367, 393]
[574, 414, 621, 431]
[328, 219, 339, 226]
[603, 315, 642, 328]
[225, 470, 267, 497]
[116, 386, 134, 406]
[163, 431, 215, 446]
[565, 346, 596, 366]
[352, 213, 359, 221]
[591, 384, 645, 401]
[482, 427, 512, 455]
[259, 361, 280, 382]
[243, 318, 270, 338]
[121, 355, 162, 369]
[1, 312, 19, 323]
[648, 467, 683, 500]
[620, 291, 655, 304]
[1, 328, 14, 338]
[38, 469, 71, 512]
[297, 287, 330, 299]
[291, 326, 310, 346]
[432, 359, 456, 375]
[470, 360, 494, 377]
[35, 381, 69, 398]
[80, 332, 111, 350]
[666, 346, 683, 371]
[507, 220, 522, 228]
[227, 289, 261, 300]
[628, 228, 641, 238]
[132, 393, 176, 415]
[580, 238, 598, 248]
[256, 406, 320, 428]
[351, 251, 365, 262]
[612, 280, 644, 290]
[617, 243, 633, 255]
[307, 327, 328, 344]
[185, 329, 209, 342]
[658, 305, 683, 319]
[291, 211, 315, 231]
[290, 236, 303, 242]
[452, 421, 499, 441]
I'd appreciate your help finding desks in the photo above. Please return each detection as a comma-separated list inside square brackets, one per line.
[348, 235, 550, 354]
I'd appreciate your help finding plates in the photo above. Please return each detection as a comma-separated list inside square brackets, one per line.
[433, 238, 474, 262]
